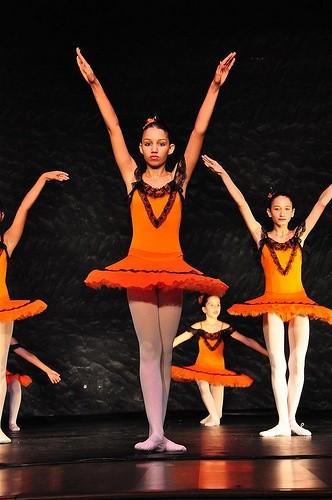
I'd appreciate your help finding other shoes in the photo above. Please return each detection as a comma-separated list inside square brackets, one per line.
[164, 442, 186, 452]
[134, 433, 166, 452]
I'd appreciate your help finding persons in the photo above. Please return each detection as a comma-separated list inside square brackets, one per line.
[76, 47, 236, 451]
[172, 292, 269, 427]
[201, 154, 332, 437]
[6, 337, 61, 432]
[0, 171, 69, 443]
